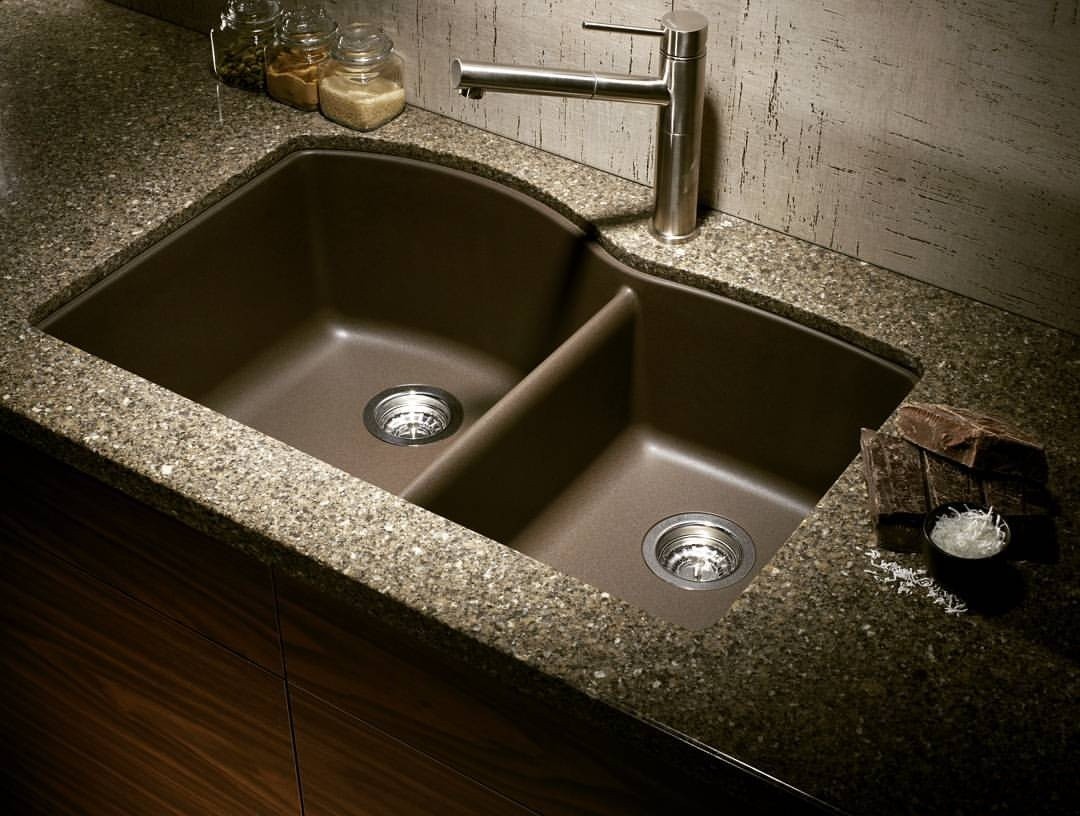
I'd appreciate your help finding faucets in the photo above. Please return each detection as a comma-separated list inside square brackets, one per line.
[449, 10, 712, 244]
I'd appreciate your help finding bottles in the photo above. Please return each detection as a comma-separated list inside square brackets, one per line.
[318, 18, 407, 133]
[263, 4, 341, 113]
[210, 0, 285, 93]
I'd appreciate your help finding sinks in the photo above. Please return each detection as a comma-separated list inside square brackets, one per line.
[28, 127, 634, 500]
[400, 275, 929, 633]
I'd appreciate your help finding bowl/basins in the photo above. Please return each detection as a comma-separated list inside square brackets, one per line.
[922, 500, 1012, 584]
